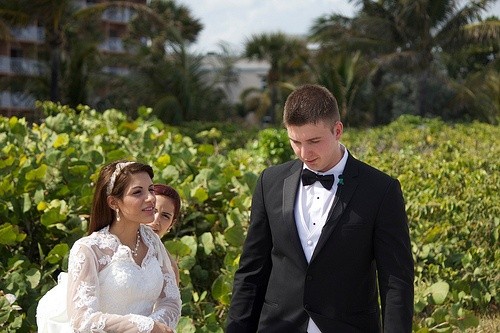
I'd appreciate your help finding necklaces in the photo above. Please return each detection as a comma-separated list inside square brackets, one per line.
[130, 229, 141, 256]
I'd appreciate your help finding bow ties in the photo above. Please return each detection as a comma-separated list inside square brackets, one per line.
[299, 170, 336, 191]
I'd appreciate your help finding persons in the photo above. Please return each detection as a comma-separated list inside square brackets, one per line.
[65, 160, 183, 333]
[220, 84, 414, 332]
[36, 181, 180, 333]
